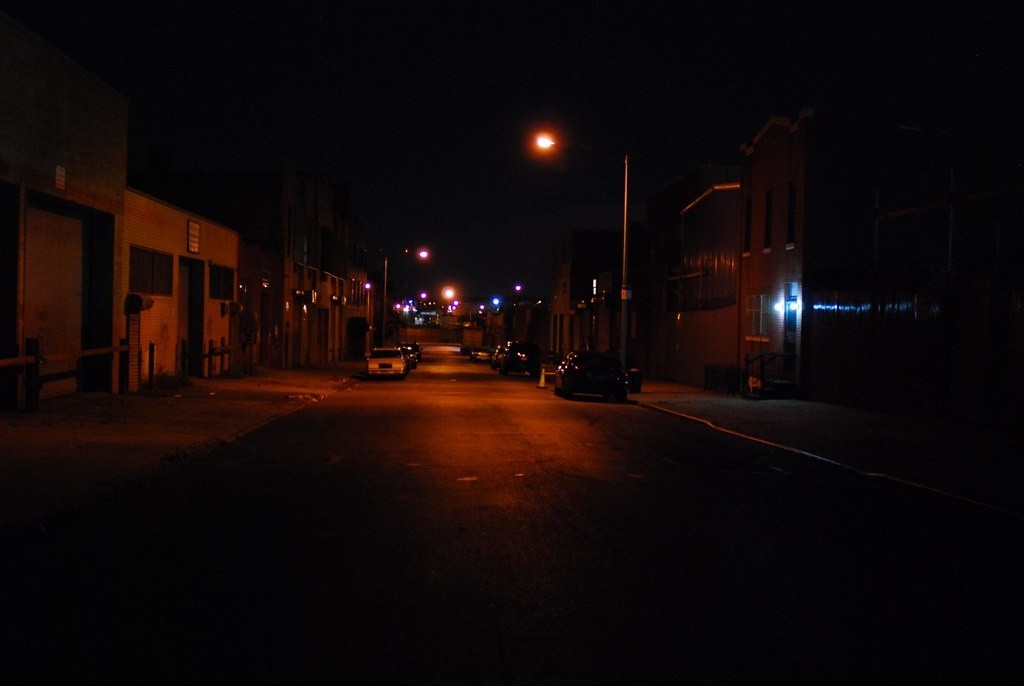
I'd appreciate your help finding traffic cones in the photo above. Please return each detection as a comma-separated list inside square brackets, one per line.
[536, 368, 548, 389]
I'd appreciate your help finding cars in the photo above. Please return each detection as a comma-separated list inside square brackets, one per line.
[553, 349, 628, 403]
[397, 340, 423, 369]
[470, 346, 493, 363]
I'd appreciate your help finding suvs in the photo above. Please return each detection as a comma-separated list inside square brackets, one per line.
[490, 340, 541, 378]
[368, 347, 409, 379]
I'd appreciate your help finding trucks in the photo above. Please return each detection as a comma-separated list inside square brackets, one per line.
[461, 328, 484, 357]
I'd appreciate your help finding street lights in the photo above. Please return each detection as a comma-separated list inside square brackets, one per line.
[530, 133, 628, 372]
[382, 248, 429, 345]
